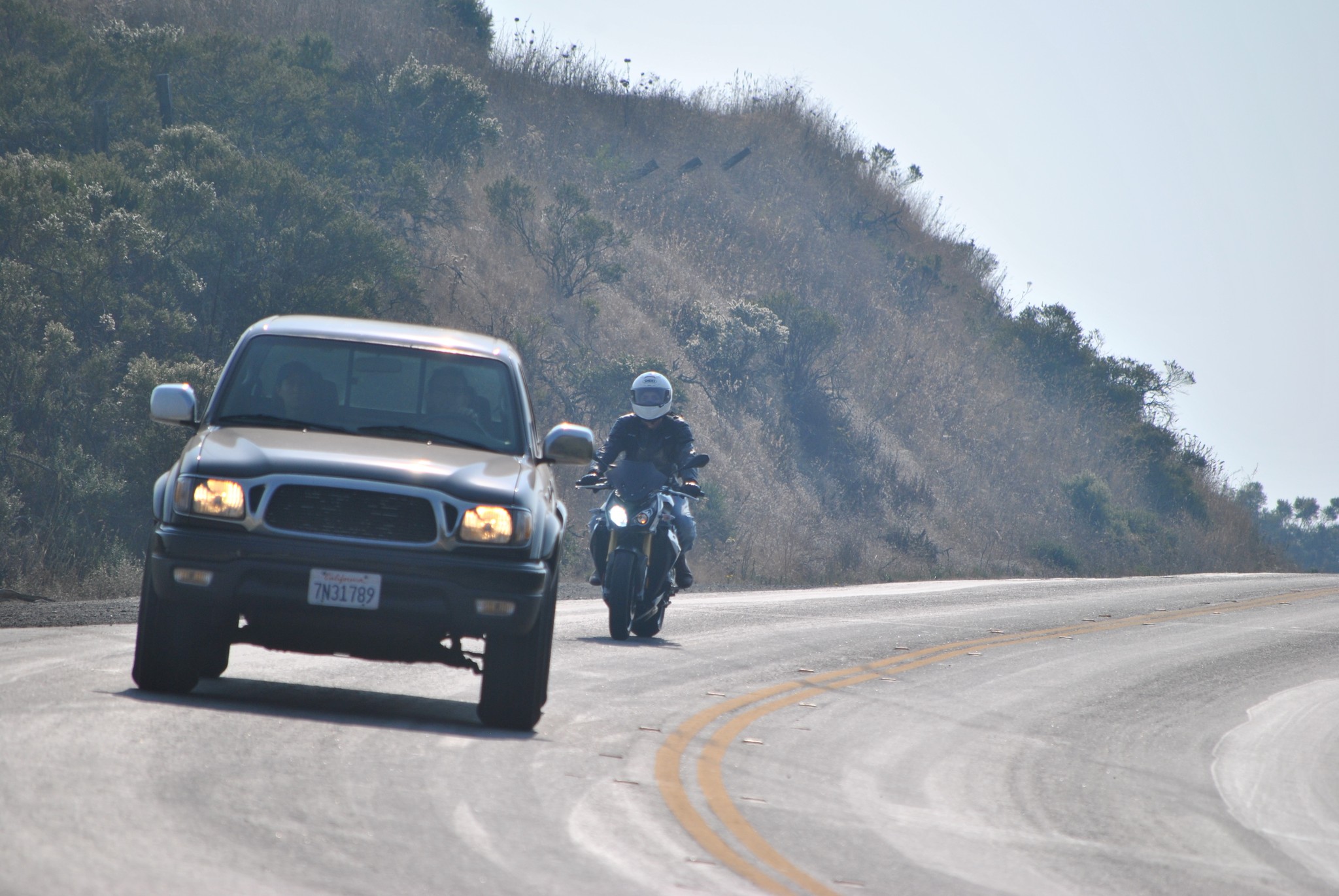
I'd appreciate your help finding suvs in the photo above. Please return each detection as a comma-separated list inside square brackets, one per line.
[130, 309, 597, 728]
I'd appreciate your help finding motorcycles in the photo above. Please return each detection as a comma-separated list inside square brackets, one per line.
[574, 452, 711, 642]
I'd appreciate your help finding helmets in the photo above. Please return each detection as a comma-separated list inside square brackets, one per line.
[630, 372, 673, 421]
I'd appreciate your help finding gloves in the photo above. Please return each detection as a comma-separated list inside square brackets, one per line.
[579, 472, 600, 494]
[680, 480, 701, 499]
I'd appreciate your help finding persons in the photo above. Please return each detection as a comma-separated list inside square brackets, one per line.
[575, 372, 702, 589]
[413, 367, 499, 442]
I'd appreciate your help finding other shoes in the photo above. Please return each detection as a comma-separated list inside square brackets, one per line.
[589, 569, 602, 586]
[673, 555, 693, 588]
